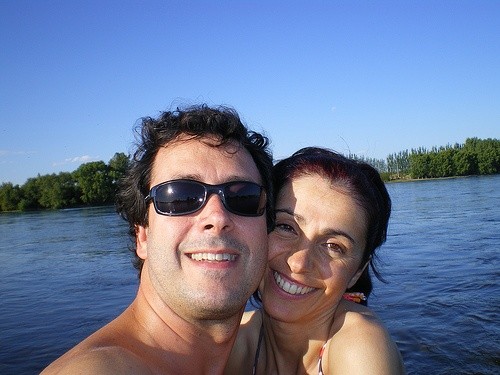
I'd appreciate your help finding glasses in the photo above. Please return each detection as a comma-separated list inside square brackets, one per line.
[144, 179, 269, 217]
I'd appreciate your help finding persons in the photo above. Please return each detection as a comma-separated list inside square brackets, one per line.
[224, 147, 407, 375]
[38, 103, 276, 375]
